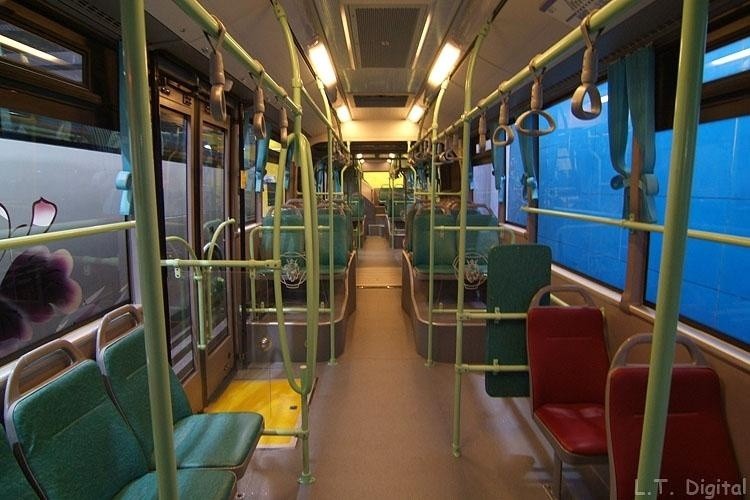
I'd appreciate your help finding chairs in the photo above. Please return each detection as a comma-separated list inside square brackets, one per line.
[255, 183, 520, 311]
[525, 284, 615, 500]
[1, 301, 266, 500]
[603, 328, 750, 499]
